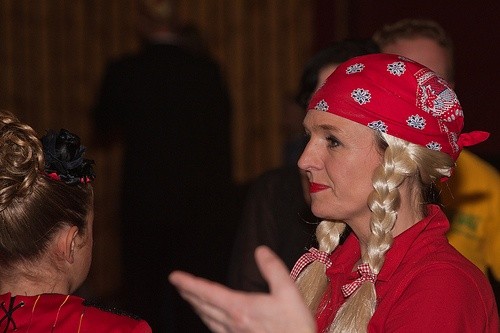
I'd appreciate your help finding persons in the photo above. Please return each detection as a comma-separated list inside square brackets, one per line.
[0, 109, 154, 333]
[91, 0, 500, 333]
